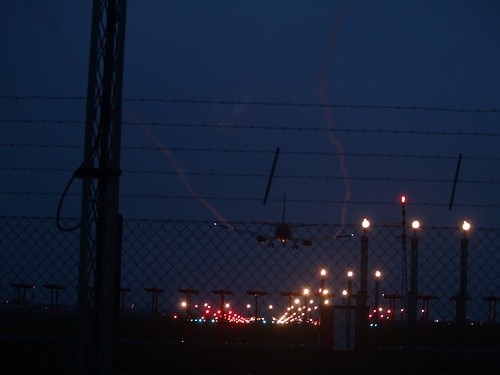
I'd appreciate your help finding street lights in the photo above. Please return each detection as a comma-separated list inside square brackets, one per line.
[172, 267, 428, 328]
[456, 221, 470, 321]
[408, 221, 421, 323]
[358, 219, 368, 315]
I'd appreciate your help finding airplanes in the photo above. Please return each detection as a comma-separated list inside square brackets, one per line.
[212, 192, 354, 250]
